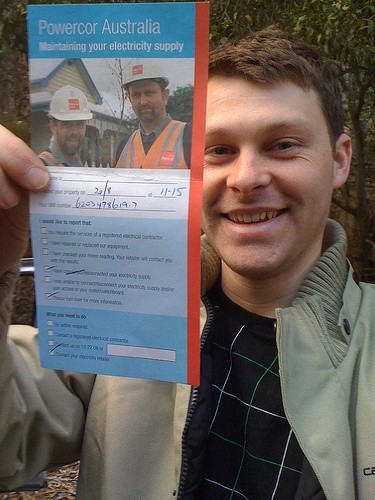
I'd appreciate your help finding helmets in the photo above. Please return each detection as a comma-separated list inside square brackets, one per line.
[48, 86, 93, 121]
[120, 62, 169, 91]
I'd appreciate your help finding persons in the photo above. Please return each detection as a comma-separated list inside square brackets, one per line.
[115, 58, 191, 170]
[0, 29, 375, 500]
[36, 85, 93, 167]
